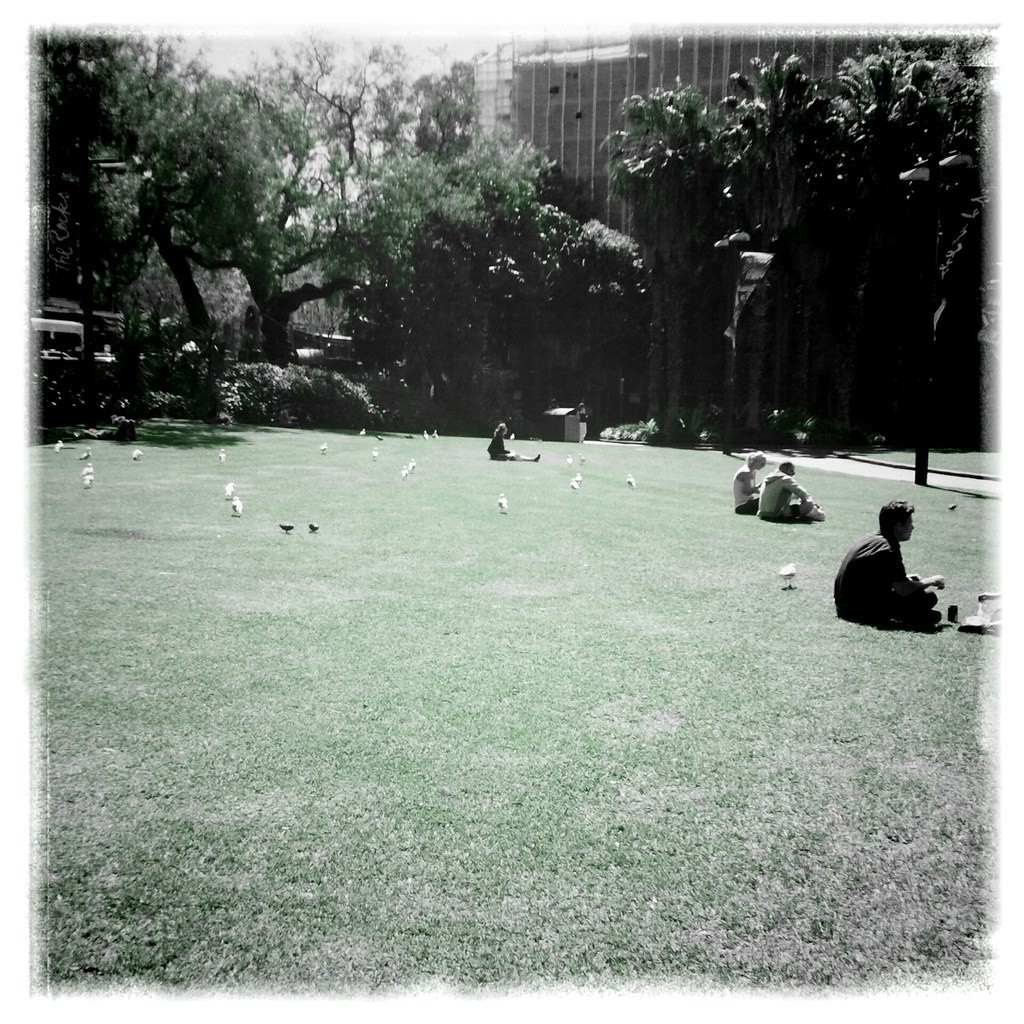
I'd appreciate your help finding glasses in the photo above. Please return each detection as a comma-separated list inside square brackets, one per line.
[791, 470, 796, 475]
[757, 460, 765, 466]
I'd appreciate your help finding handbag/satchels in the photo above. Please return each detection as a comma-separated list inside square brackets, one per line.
[799, 499, 826, 521]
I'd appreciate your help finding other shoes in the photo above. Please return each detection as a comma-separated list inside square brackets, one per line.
[534, 454, 540, 462]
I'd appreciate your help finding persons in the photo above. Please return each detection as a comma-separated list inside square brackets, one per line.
[487, 423, 540, 462]
[733, 450, 815, 522]
[100, 416, 136, 441]
[834, 499, 945, 631]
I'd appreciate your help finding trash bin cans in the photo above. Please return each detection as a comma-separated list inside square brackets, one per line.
[542, 405, 580, 443]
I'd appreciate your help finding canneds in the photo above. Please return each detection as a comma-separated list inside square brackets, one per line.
[947, 604, 958, 622]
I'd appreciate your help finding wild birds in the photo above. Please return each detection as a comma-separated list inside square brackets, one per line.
[371, 447, 379, 462]
[51, 438, 144, 490]
[422, 428, 439, 441]
[566, 452, 589, 468]
[216, 446, 228, 465]
[318, 442, 328, 454]
[776, 561, 796, 595]
[624, 473, 638, 489]
[357, 427, 367, 437]
[949, 501, 960, 512]
[277, 519, 322, 534]
[495, 493, 510, 513]
[223, 481, 244, 520]
[399, 459, 416, 481]
[569, 472, 583, 495]
